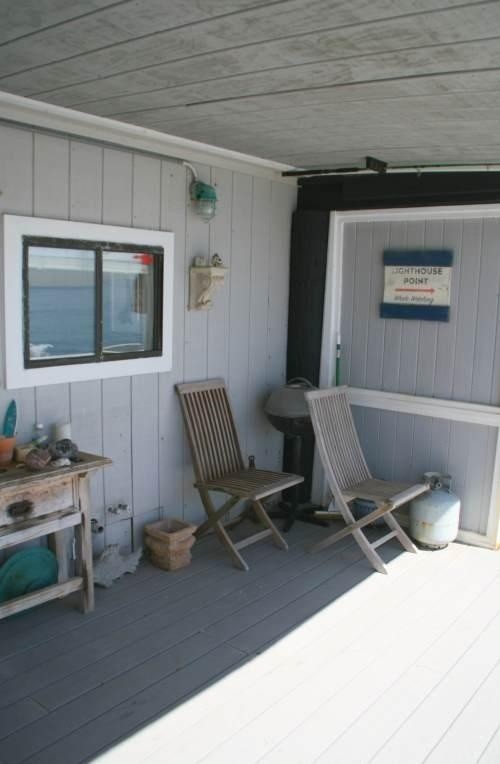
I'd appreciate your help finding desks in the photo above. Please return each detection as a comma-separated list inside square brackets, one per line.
[0, 445, 111, 621]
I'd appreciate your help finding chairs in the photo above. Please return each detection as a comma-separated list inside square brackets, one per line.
[302, 385, 429, 575]
[175, 375, 307, 570]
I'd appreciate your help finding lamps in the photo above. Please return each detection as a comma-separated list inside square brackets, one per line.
[188, 179, 218, 225]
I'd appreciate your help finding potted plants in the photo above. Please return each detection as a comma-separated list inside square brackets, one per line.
[0, 399, 17, 466]
